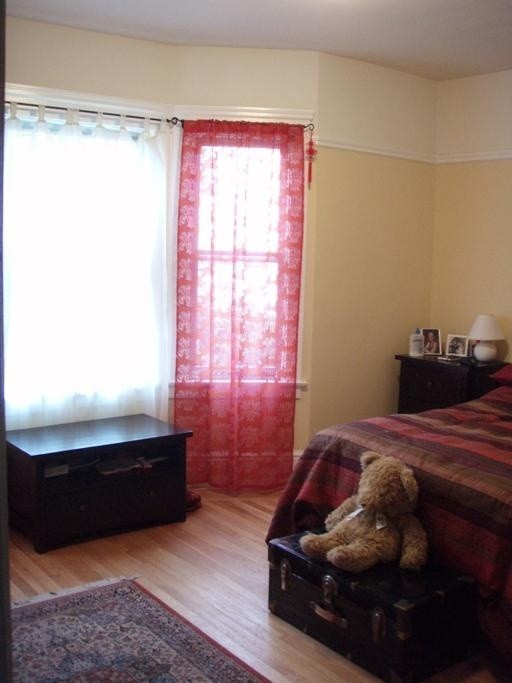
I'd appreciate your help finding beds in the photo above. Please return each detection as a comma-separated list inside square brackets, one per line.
[263, 383, 512, 682]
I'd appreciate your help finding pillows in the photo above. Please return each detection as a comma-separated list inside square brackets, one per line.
[490, 359, 511, 389]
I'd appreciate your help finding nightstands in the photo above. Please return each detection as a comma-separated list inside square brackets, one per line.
[393, 353, 509, 418]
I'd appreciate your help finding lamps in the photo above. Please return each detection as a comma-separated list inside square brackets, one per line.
[466, 312, 507, 362]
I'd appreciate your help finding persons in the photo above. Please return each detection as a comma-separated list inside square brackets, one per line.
[424, 331, 438, 353]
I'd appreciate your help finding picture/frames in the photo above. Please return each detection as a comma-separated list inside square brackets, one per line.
[445, 333, 470, 357]
[420, 326, 443, 355]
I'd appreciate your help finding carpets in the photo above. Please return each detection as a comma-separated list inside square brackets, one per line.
[9, 570, 274, 682]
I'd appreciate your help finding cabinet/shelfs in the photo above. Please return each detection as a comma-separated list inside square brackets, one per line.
[2, 410, 195, 555]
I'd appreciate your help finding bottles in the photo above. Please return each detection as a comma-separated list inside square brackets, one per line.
[408, 327, 424, 356]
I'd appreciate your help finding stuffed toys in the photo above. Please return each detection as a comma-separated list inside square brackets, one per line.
[299, 451, 430, 573]
